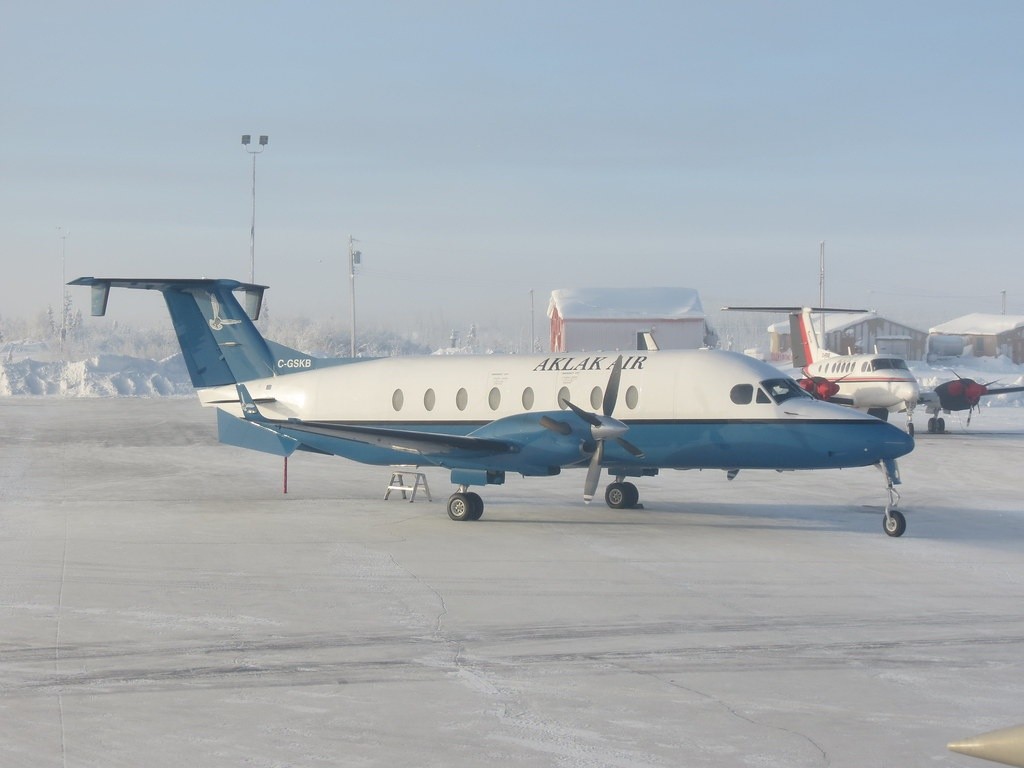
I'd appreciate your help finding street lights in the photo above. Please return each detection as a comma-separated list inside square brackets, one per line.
[528, 288, 535, 353]
[240, 133, 270, 285]
[347, 234, 361, 358]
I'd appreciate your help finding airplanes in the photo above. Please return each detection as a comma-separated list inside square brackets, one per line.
[63, 273, 918, 540]
[637, 306, 1024, 437]
